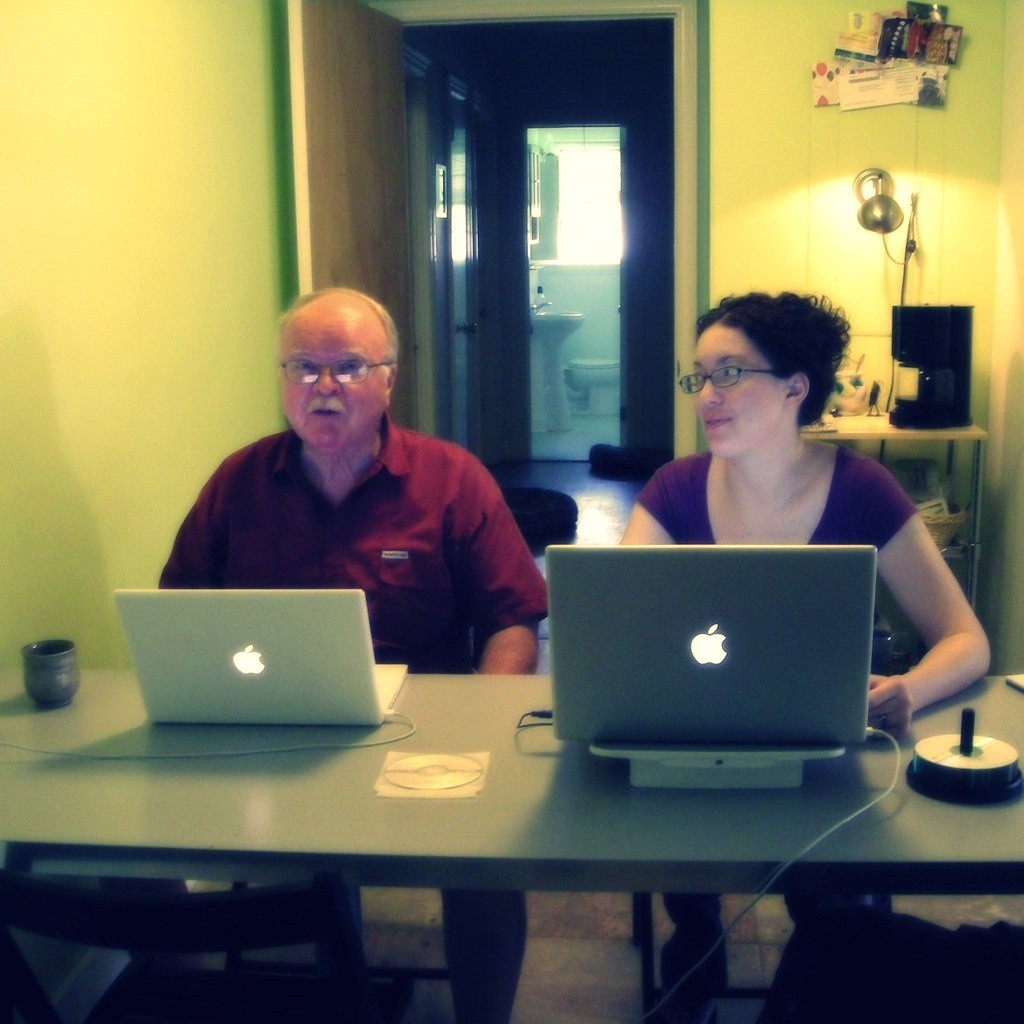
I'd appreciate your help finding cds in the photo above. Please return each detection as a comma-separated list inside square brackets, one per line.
[913, 734, 1020, 793]
[385, 754, 484, 790]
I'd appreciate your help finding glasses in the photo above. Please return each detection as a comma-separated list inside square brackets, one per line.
[680, 366, 774, 396]
[282, 359, 392, 384]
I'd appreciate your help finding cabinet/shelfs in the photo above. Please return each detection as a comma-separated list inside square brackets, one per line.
[802, 412, 989, 617]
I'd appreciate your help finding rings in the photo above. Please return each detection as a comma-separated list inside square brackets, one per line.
[881, 714, 888, 730]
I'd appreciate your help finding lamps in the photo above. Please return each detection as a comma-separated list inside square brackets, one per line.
[852, 168, 919, 307]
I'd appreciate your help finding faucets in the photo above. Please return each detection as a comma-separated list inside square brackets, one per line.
[537, 301, 553, 309]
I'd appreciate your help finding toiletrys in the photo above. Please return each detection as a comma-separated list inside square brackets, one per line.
[535, 285, 545, 303]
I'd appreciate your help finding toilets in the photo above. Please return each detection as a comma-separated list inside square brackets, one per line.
[564, 357, 621, 416]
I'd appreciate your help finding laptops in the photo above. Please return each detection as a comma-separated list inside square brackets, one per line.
[543, 545, 877, 747]
[114, 588, 405, 727]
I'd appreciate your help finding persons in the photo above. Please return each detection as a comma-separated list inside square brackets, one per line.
[156, 283, 556, 1024]
[619, 288, 993, 1023]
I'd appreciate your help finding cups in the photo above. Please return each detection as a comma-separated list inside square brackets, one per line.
[831, 371, 866, 416]
[23, 640, 79, 706]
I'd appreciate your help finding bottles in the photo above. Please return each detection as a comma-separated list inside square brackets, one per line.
[536, 286, 546, 315]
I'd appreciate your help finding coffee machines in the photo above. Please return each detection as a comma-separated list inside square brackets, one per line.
[889, 306, 972, 428]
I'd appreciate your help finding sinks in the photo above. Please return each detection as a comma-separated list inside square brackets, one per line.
[531, 309, 585, 345]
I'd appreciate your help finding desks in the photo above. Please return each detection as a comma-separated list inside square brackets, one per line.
[0, 669, 1024, 1024]
